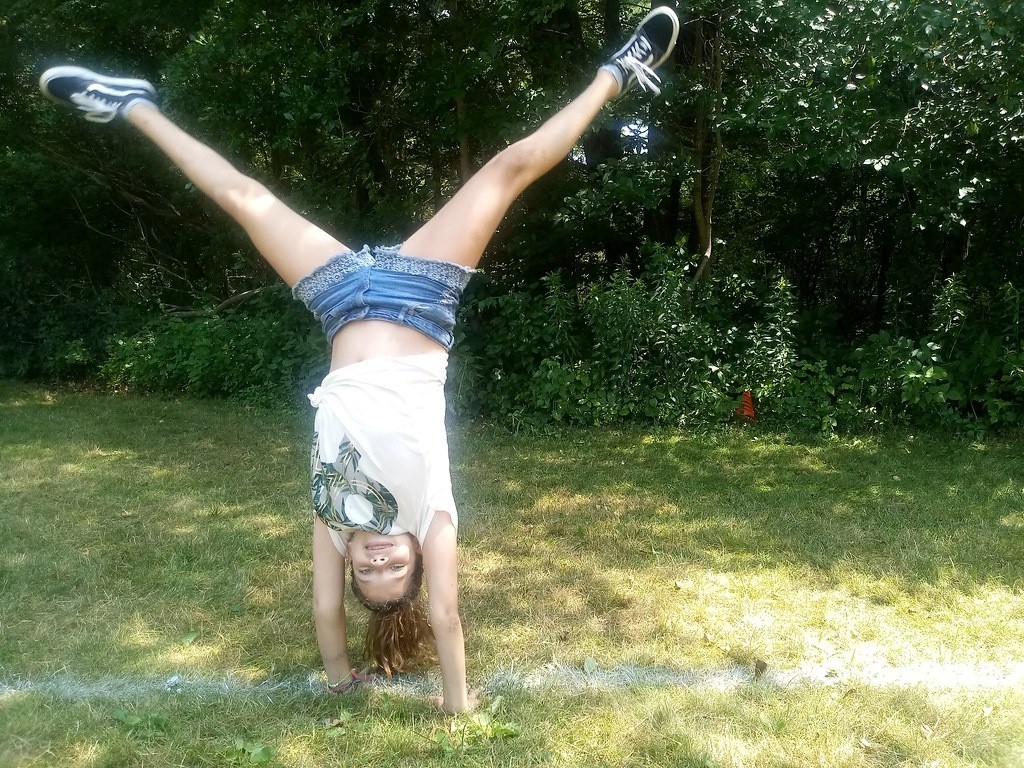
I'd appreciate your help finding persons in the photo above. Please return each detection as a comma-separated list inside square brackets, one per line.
[39, 6, 679, 716]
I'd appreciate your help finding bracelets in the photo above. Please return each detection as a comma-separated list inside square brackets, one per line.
[327, 672, 359, 691]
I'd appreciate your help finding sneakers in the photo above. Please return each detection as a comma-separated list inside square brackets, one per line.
[599, 6, 678, 102]
[39, 66, 157, 124]
